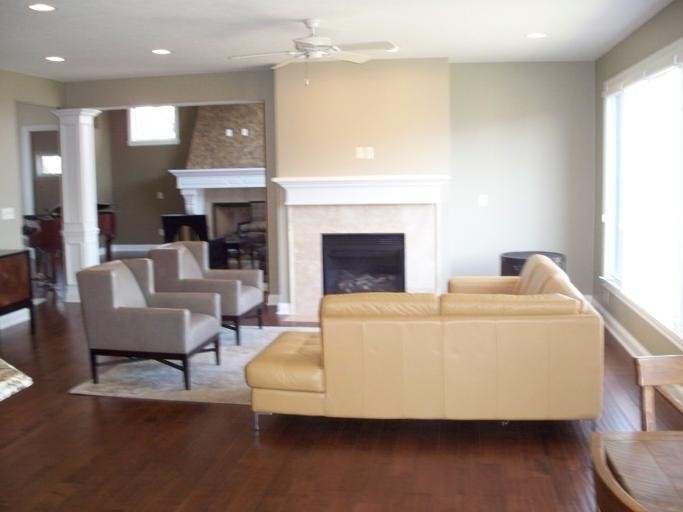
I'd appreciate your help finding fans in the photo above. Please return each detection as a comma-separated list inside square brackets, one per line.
[229, 20, 394, 71]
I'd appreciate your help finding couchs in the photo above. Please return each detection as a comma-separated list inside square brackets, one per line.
[246, 279, 606, 431]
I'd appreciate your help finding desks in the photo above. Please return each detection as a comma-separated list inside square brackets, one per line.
[0, 249, 37, 335]
[502, 251, 565, 277]
[605, 432, 683, 512]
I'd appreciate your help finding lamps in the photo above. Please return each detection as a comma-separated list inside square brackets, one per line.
[306, 51, 326, 58]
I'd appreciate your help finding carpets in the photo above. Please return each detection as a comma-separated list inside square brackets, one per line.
[67, 325, 322, 405]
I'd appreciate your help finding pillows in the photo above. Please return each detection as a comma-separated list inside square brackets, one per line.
[320, 252, 587, 315]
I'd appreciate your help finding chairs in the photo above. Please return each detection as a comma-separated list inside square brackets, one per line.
[152, 240, 264, 346]
[77, 258, 221, 390]
[592, 432, 649, 512]
[634, 355, 683, 431]
